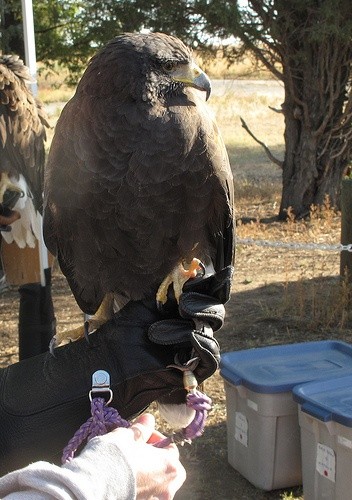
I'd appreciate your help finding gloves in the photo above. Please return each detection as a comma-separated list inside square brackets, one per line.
[0, 265, 235, 479]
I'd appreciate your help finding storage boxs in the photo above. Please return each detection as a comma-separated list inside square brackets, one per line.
[291, 371, 352, 500]
[218, 340, 352, 493]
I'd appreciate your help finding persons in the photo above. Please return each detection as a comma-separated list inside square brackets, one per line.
[0, 413, 186, 500]
